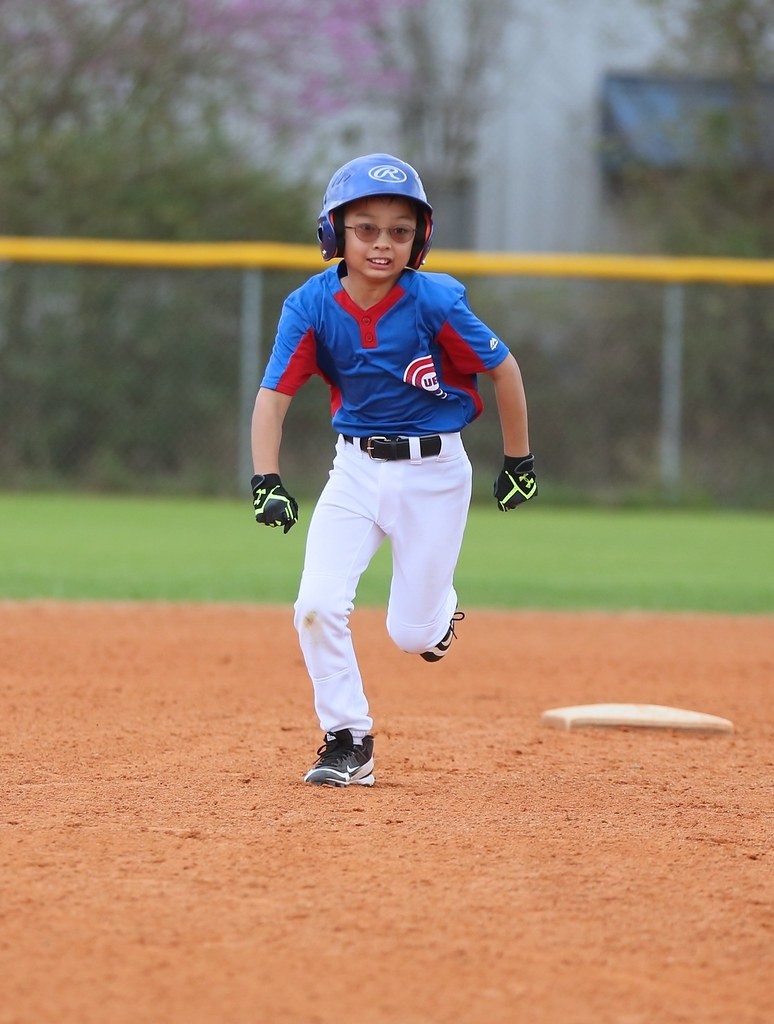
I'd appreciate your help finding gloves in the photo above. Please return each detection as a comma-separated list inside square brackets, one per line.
[493, 453, 538, 512]
[250, 474, 298, 534]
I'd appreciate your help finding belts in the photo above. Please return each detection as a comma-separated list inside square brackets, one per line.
[343, 435, 442, 462]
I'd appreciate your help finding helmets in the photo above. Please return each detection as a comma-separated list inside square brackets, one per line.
[315, 153, 434, 269]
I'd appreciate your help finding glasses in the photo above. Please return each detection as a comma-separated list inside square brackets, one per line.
[340, 222, 419, 244]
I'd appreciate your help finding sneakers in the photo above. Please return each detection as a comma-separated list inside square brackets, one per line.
[420, 604, 465, 663]
[304, 728, 375, 788]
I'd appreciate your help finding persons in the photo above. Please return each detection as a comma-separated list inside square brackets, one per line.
[250, 155, 539, 788]
